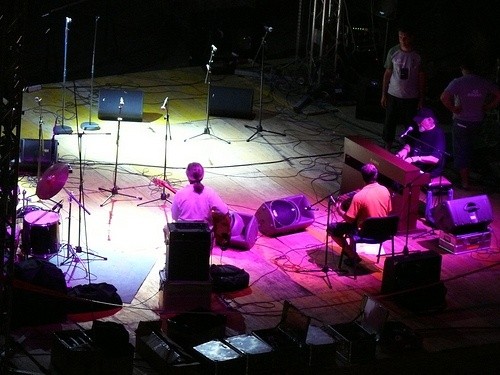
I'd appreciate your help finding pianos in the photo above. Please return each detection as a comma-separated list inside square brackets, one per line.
[339, 134, 431, 236]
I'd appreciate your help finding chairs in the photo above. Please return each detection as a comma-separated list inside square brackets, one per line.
[338, 215, 399, 280]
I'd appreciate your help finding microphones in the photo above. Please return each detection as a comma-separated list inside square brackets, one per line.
[401, 126, 413, 138]
[263, 26, 273, 32]
[211, 44, 217, 51]
[120, 97, 125, 105]
[161, 97, 168, 110]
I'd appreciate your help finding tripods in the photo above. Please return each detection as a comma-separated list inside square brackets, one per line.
[97, 105, 142, 207]
[138, 105, 174, 206]
[379, 171, 426, 257]
[57, 80, 108, 283]
[244, 31, 287, 142]
[183, 52, 231, 144]
[294, 188, 349, 290]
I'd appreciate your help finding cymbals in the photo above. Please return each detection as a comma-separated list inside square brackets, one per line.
[36, 162, 69, 199]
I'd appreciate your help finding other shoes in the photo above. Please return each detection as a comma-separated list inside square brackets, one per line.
[344, 256, 361, 264]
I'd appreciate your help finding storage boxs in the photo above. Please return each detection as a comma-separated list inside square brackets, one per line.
[135, 319, 200, 375]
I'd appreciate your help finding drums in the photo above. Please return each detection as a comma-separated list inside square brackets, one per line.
[23, 210, 61, 258]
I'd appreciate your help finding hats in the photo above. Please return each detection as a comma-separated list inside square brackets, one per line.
[413, 109, 434, 121]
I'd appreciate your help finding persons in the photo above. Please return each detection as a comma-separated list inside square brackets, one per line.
[171, 162, 230, 229]
[395, 108, 446, 192]
[326, 164, 392, 265]
[440, 59, 500, 192]
[381, 24, 424, 152]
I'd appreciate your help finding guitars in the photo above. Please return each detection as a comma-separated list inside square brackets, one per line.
[152, 178, 231, 247]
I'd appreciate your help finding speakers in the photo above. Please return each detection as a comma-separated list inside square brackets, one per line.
[228, 213, 259, 249]
[162, 221, 212, 283]
[98, 87, 144, 121]
[435, 193, 493, 232]
[356, 81, 389, 122]
[254, 193, 314, 237]
[208, 85, 256, 120]
[17, 139, 59, 172]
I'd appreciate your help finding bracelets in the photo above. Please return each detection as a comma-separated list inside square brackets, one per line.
[419, 156, 421, 160]
